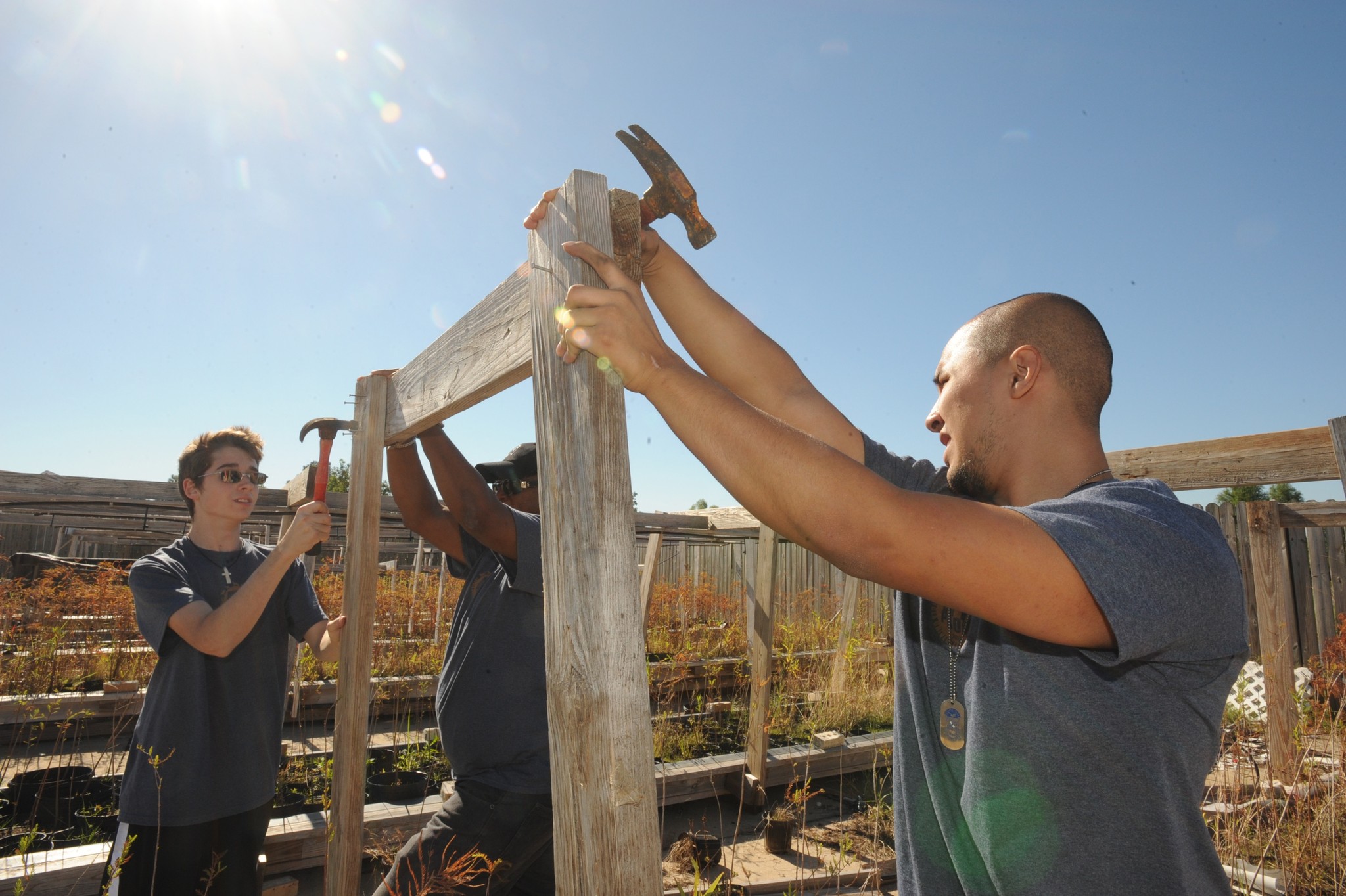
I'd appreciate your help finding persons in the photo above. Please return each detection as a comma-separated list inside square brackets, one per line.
[100, 423, 346, 896]
[371, 370, 556, 896]
[525, 189, 1249, 895]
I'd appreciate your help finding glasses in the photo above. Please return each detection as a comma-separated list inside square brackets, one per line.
[491, 479, 538, 496]
[191, 469, 268, 485]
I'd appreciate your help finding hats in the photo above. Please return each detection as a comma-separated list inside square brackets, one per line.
[475, 442, 537, 484]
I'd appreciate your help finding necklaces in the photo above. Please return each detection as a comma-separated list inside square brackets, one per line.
[186, 534, 244, 583]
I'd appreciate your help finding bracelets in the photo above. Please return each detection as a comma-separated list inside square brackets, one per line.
[396, 437, 416, 448]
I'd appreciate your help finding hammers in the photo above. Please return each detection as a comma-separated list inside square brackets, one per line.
[300, 419, 359, 554]
[614, 125, 718, 250]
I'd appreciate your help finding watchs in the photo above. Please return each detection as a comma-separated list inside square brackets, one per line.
[416, 422, 444, 438]
